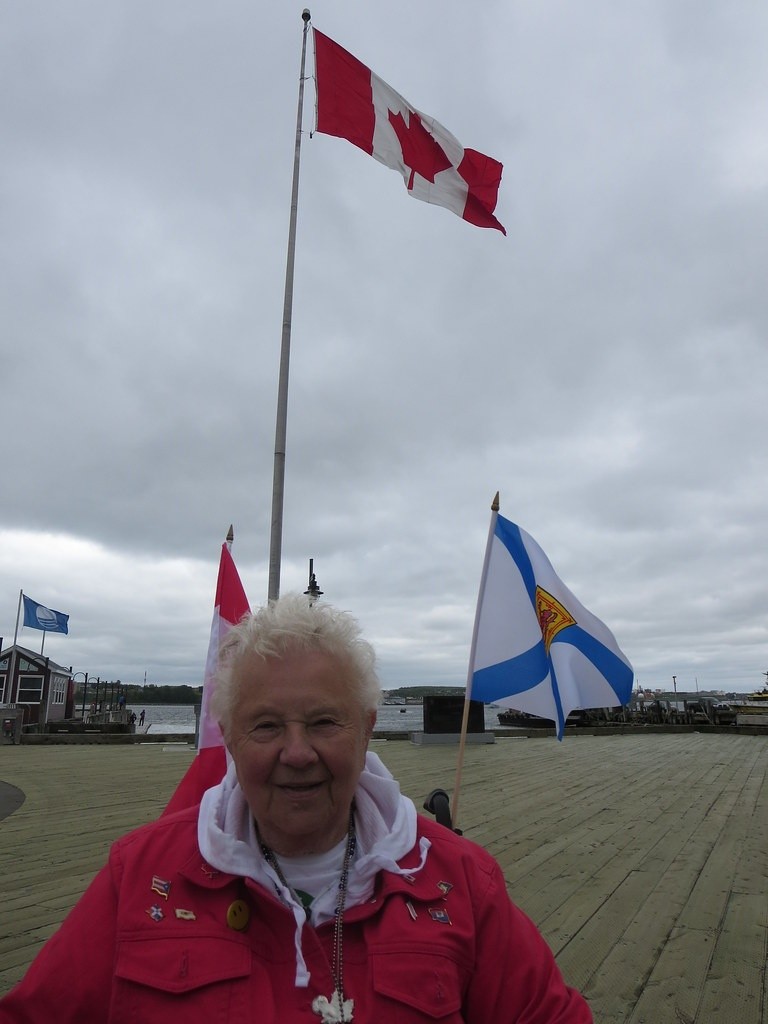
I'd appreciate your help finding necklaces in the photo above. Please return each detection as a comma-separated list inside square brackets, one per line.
[252, 809, 355, 1023]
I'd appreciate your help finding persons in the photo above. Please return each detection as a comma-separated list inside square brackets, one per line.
[0, 591, 593, 1024]
[118, 694, 147, 727]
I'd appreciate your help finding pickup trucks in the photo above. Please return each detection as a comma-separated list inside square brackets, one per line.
[687, 697, 721, 712]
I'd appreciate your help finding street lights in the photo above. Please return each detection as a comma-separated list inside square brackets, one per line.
[73, 672, 89, 717]
[89, 677, 114, 715]
[27, 657, 49, 724]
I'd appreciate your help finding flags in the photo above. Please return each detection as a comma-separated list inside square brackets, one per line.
[162, 542, 251, 815]
[310, 29, 504, 237]
[22, 593, 70, 635]
[470, 514, 635, 744]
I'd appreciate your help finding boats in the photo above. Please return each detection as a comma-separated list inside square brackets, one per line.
[497, 713, 555, 728]
[728, 693, 768, 713]
